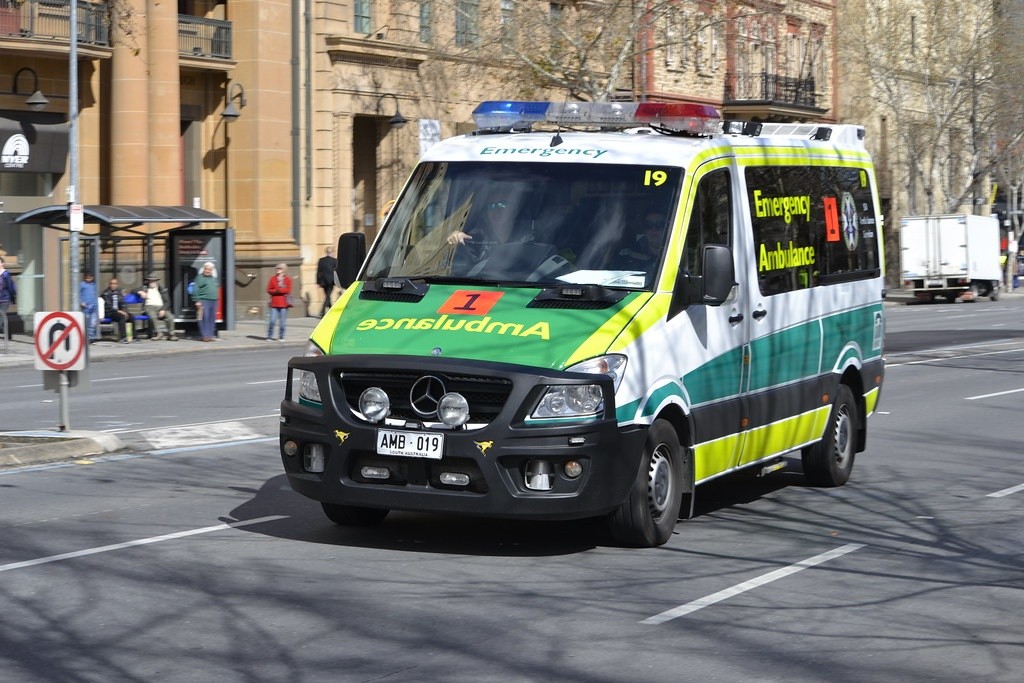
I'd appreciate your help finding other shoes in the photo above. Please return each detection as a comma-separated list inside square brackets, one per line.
[200, 336, 215, 342]
[134, 338, 141, 343]
[120, 338, 128, 344]
[266, 339, 271, 342]
[151, 333, 164, 340]
[167, 334, 178, 341]
[278, 339, 284, 342]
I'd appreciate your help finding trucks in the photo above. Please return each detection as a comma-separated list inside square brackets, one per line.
[898, 214, 1002, 304]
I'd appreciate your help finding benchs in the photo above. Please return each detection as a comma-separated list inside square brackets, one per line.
[98, 292, 160, 340]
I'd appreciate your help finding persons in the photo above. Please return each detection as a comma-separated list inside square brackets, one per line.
[603, 209, 687, 289]
[0, 257, 17, 340]
[80, 270, 98, 345]
[445, 188, 539, 278]
[187, 262, 220, 341]
[316, 246, 338, 316]
[265, 263, 292, 341]
[130, 272, 179, 341]
[100, 279, 140, 344]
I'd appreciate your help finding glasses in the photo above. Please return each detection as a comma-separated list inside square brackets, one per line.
[487, 202, 511, 209]
[644, 220, 665, 232]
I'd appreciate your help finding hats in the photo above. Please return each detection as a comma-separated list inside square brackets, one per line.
[145, 273, 160, 280]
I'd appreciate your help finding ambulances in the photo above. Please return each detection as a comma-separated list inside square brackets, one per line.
[279, 98, 887, 549]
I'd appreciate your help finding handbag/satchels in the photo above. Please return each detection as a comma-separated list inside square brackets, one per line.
[286, 295, 295, 307]
[187, 282, 195, 294]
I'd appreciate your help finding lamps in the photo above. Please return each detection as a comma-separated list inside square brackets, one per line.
[219, 83, 246, 122]
[13, 67, 50, 111]
[375, 93, 412, 129]
